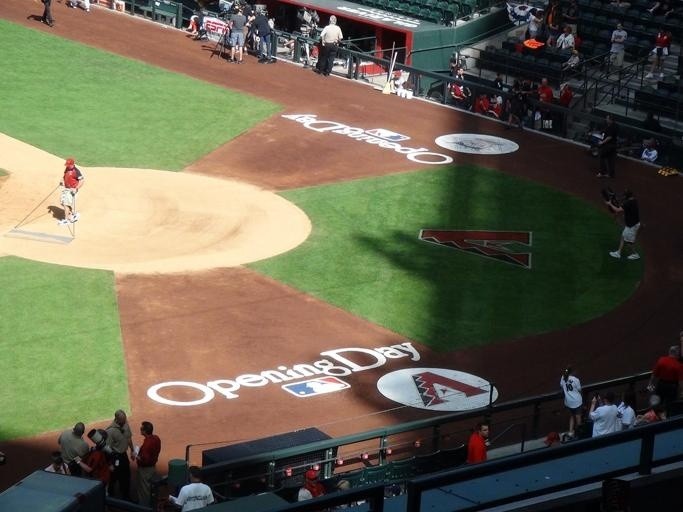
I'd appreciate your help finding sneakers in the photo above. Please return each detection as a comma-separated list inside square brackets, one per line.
[609, 251, 620, 259]
[627, 253, 639, 260]
[69, 214, 79, 222]
[59, 219, 67, 223]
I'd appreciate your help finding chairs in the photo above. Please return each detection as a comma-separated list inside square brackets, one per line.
[357, 0, 498, 27]
[633, 70, 682, 106]
[575, 1, 681, 61]
[476, 36, 574, 90]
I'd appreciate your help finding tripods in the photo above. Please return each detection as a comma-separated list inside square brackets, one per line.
[210, 24, 233, 58]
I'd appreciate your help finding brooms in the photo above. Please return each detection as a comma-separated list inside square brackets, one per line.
[381, 51, 398, 94]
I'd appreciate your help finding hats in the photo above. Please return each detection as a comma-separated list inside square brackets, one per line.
[64, 159, 73, 166]
